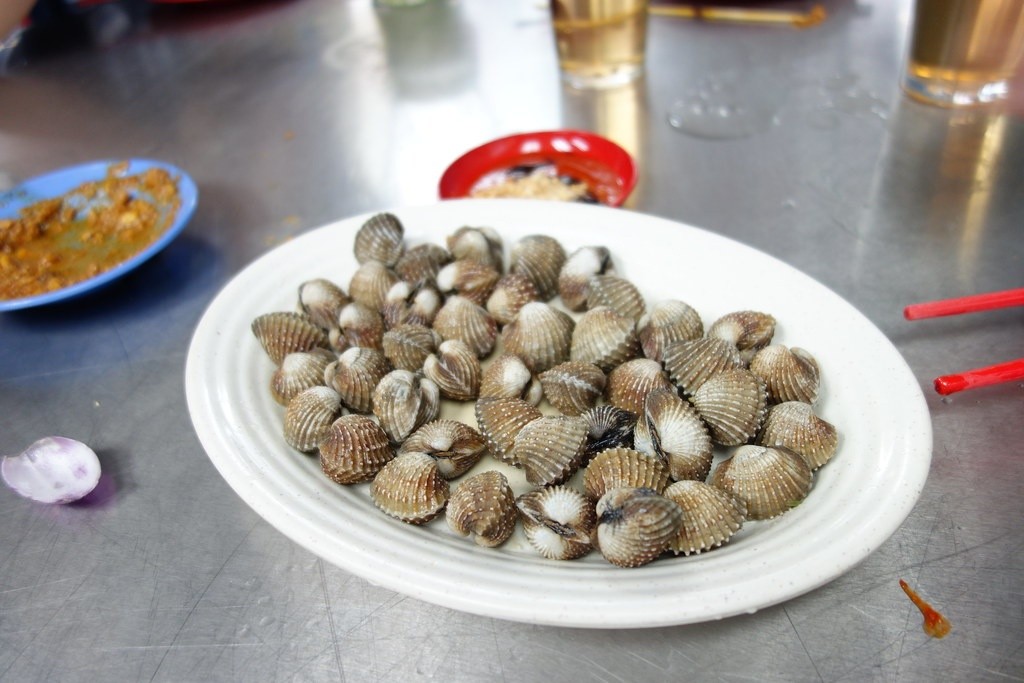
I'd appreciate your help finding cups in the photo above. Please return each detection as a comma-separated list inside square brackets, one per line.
[902, 0, 1024, 108]
[550, 0, 646, 91]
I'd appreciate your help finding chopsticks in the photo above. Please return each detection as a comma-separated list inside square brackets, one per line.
[903, 289, 1024, 395]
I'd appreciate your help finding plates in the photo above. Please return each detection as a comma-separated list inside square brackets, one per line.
[184, 199, 931, 628]
[0, 159, 198, 312]
[440, 131, 638, 208]
